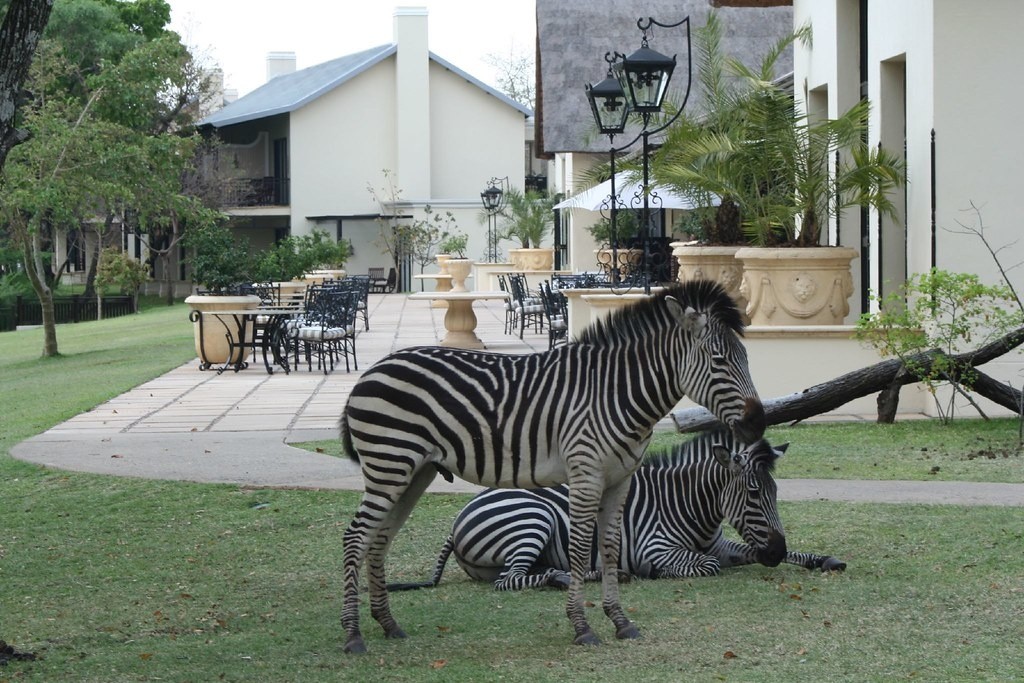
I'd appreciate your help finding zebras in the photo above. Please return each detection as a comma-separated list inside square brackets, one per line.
[339, 280, 847, 653]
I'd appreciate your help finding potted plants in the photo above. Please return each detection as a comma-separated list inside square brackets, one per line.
[478, 185, 559, 270]
[563, 6, 911, 326]
[435, 232, 472, 291]
[178, 218, 262, 365]
[252, 225, 353, 306]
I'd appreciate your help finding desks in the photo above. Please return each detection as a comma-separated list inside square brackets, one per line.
[410, 291, 509, 349]
[264, 299, 305, 303]
[269, 293, 306, 297]
[202, 311, 308, 374]
[415, 274, 474, 292]
[258, 306, 304, 308]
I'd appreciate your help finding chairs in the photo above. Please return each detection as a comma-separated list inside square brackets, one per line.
[196, 275, 372, 375]
[498, 272, 659, 350]
[369, 268, 396, 294]
